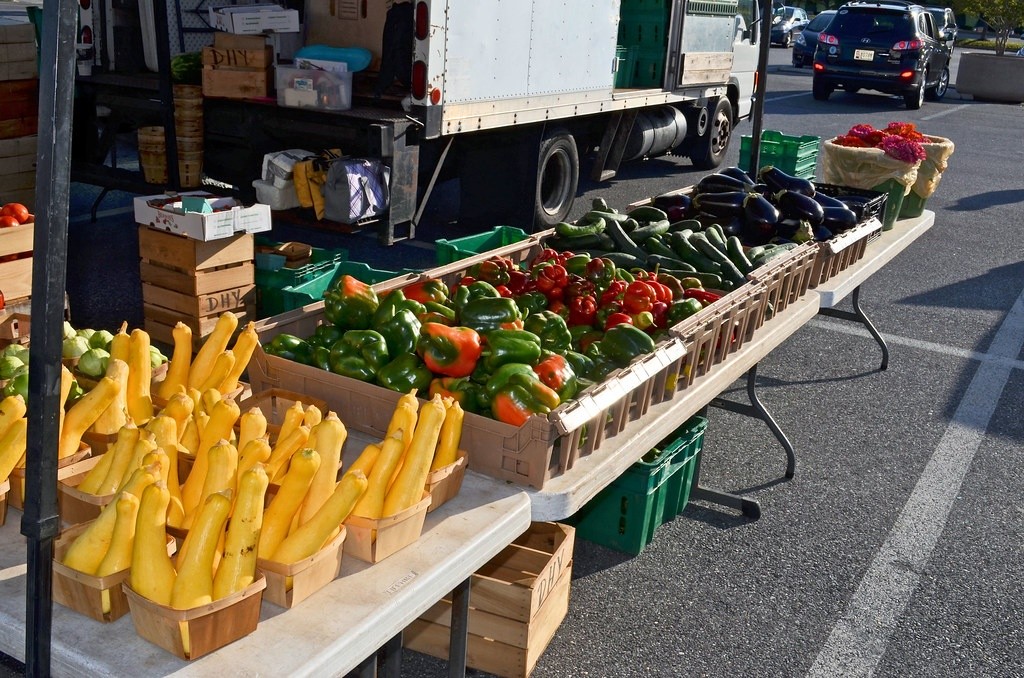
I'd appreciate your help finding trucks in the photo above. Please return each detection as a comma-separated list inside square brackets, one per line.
[73, 0, 761, 246]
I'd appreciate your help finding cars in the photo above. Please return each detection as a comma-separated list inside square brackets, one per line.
[792, 10, 839, 68]
[759, 5, 811, 49]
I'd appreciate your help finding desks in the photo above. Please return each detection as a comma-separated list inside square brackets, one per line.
[530, 287, 822, 526]
[811, 208, 935, 371]
[0, 391, 531, 678]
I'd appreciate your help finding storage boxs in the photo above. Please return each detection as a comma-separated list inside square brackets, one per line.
[434, 225, 528, 267]
[0, 10, 39, 340]
[557, 414, 708, 556]
[282, 260, 429, 312]
[738, 129, 822, 182]
[611, 0, 672, 88]
[134, 190, 272, 356]
[254, 238, 351, 316]
[204, 0, 394, 110]
[402, 521, 576, 678]
[2, 335, 468, 659]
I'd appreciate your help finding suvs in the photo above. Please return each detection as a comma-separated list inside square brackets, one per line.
[923, 5, 958, 66]
[812, 0, 951, 110]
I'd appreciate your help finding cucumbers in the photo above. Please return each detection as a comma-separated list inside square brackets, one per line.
[549, 197, 802, 290]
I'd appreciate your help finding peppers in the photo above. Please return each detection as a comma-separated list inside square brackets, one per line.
[264, 248, 737, 451]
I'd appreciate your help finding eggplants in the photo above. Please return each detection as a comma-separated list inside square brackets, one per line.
[651, 164, 877, 243]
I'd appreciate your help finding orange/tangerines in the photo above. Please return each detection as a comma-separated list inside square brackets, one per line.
[0, 201, 30, 264]
